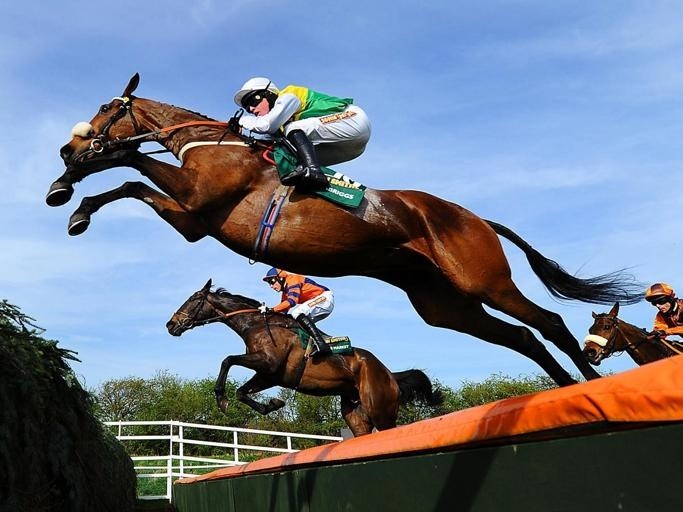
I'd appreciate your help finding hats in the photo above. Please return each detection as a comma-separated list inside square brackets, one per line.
[233, 77, 280, 107]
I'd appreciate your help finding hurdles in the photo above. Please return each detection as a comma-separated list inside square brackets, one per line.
[172, 352, 683, 511]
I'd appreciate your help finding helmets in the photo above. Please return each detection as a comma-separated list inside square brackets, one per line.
[262, 268, 287, 280]
[645, 283, 676, 302]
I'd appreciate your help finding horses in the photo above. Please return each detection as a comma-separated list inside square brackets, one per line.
[165, 278, 445, 437]
[582, 302, 683, 366]
[44, 73, 644, 387]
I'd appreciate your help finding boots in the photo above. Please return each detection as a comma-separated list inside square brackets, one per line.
[280, 129, 327, 187]
[299, 316, 332, 357]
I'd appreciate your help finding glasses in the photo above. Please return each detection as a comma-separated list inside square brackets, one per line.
[652, 295, 668, 306]
[268, 278, 279, 284]
[240, 89, 263, 113]
[274, 96, 276, 97]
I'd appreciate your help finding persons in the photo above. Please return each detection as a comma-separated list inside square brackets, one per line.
[644, 282, 682, 340]
[259, 265, 335, 359]
[227, 76, 371, 192]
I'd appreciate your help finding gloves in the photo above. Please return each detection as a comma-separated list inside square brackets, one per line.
[228, 117, 239, 135]
[650, 330, 666, 339]
[258, 305, 272, 313]
